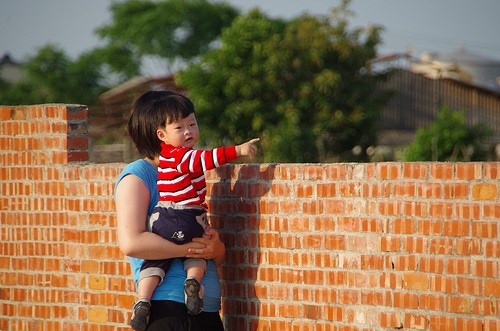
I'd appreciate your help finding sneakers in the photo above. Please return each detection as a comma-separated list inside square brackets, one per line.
[129, 298, 151, 331]
[183, 276, 204, 315]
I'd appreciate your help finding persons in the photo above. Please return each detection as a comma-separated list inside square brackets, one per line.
[130, 94, 260, 331]
[114, 91, 226, 331]
